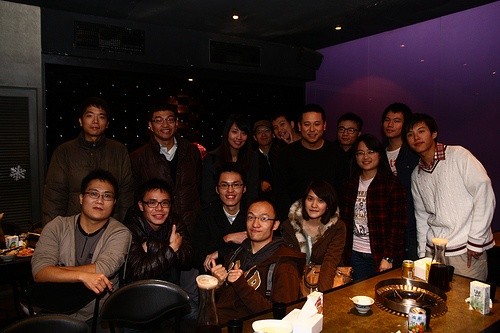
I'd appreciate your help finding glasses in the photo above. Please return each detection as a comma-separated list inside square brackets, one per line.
[337, 127, 359, 134]
[356, 149, 377, 157]
[150, 117, 175, 123]
[142, 199, 171, 208]
[246, 215, 275, 222]
[217, 182, 243, 189]
[84, 191, 115, 201]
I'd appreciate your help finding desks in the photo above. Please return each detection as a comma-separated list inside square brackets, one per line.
[222, 266, 500, 333]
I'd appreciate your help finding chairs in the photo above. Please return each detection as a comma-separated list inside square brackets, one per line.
[100, 279, 192, 333]
[0, 280, 100, 333]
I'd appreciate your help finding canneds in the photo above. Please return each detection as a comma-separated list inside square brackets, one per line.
[402, 260, 414, 281]
[408, 307, 426, 333]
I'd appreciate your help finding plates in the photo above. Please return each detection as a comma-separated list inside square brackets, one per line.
[16, 247, 35, 257]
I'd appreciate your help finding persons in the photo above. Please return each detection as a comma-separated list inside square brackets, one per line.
[280, 180, 347, 292]
[28, 96, 497, 333]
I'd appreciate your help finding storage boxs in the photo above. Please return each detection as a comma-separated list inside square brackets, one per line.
[469, 280, 491, 315]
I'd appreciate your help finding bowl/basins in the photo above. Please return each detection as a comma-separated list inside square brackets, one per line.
[352, 296, 375, 314]
[0, 249, 19, 261]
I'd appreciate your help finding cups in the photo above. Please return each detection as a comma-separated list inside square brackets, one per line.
[252, 319, 294, 333]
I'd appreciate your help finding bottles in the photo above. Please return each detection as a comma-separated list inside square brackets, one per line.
[431, 238, 447, 265]
[19, 233, 28, 247]
[227, 318, 244, 333]
[271, 302, 286, 320]
[195, 275, 222, 333]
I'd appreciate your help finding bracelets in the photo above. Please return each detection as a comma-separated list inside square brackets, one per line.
[382, 256, 393, 262]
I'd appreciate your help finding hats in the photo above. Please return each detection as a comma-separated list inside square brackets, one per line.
[254, 120, 271, 130]
